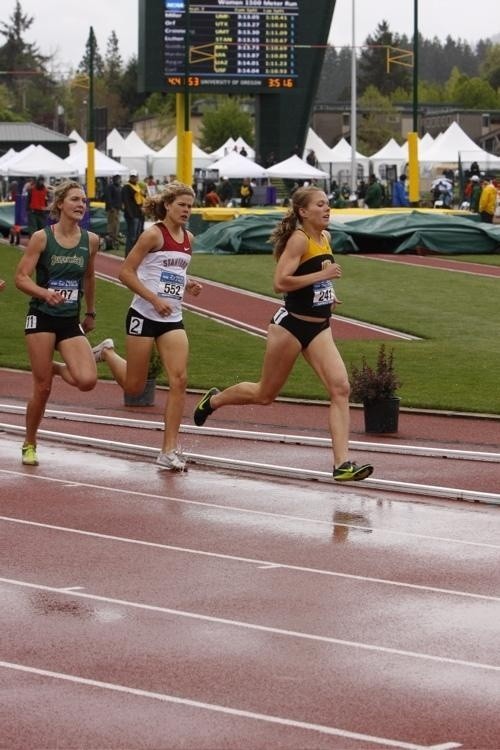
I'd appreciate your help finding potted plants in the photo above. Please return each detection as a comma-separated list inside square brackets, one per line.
[350, 344, 402, 433]
[124, 343, 162, 407]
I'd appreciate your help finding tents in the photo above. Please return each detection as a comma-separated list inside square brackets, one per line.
[0, 145, 78, 199]
[205, 150, 331, 194]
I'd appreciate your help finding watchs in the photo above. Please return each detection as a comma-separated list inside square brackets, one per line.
[85, 312, 97, 318]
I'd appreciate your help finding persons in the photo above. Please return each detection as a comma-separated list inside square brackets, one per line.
[14, 180, 101, 465]
[429, 162, 500, 223]
[290, 174, 411, 208]
[193, 187, 374, 482]
[2, 169, 145, 260]
[194, 178, 257, 207]
[93, 181, 203, 473]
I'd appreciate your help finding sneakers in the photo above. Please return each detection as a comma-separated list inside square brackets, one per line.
[92, 338, 114, 363]
[22, 440, 39, 465]
[333, 461, 373, 481]
[193, 387, 220, 426]
[157, 449, 186, 469]
